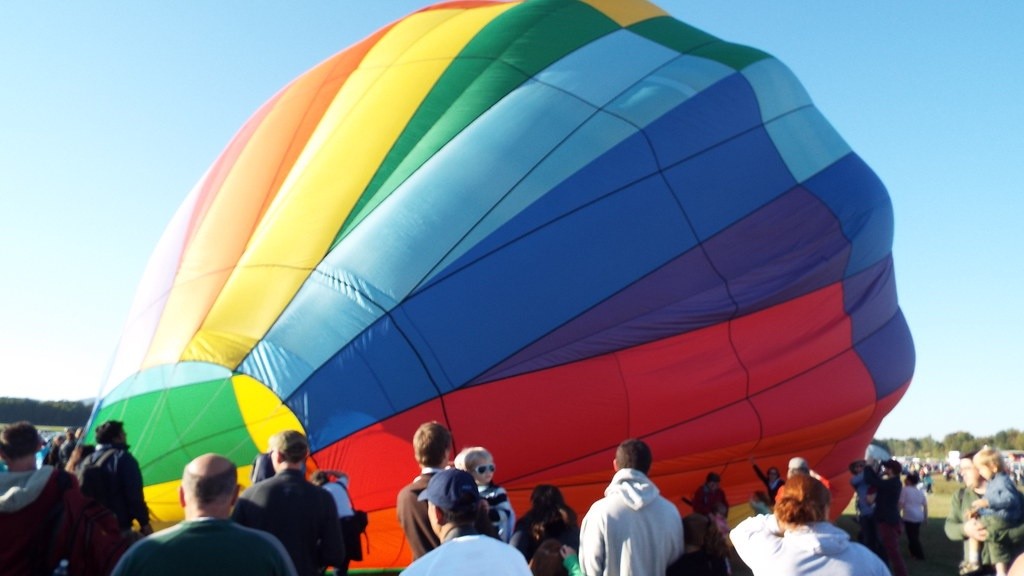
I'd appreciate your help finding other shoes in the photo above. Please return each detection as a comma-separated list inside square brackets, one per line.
[959, 561, 980, 576]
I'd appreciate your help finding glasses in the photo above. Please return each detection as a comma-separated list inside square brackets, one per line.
[769, 472, 778, 476]
[472, 464, 496, 475]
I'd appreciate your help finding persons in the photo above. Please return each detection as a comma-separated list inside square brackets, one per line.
[111, 452, 298, 576]
[578, 439, 685, 576]
[395, 421, 534, 576]
[943, 445, 1024, 576]
[510, 485, 580, 576]
[232, 431, 363, 576]
[665, 457, 891, 576]
[0, 419, 154, 575]
[880, 459, 938, 576]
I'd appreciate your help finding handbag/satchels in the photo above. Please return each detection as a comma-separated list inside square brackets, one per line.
[342, 511, 369, 534]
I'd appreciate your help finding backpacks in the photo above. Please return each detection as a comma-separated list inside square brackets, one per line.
[78, 446, 118, 515]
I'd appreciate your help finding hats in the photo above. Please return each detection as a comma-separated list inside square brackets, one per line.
[416, 467, 480, 515]
[788, 457, 809, 470]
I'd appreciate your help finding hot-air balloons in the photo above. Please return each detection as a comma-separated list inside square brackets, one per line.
[65, 0, 915, 576]
